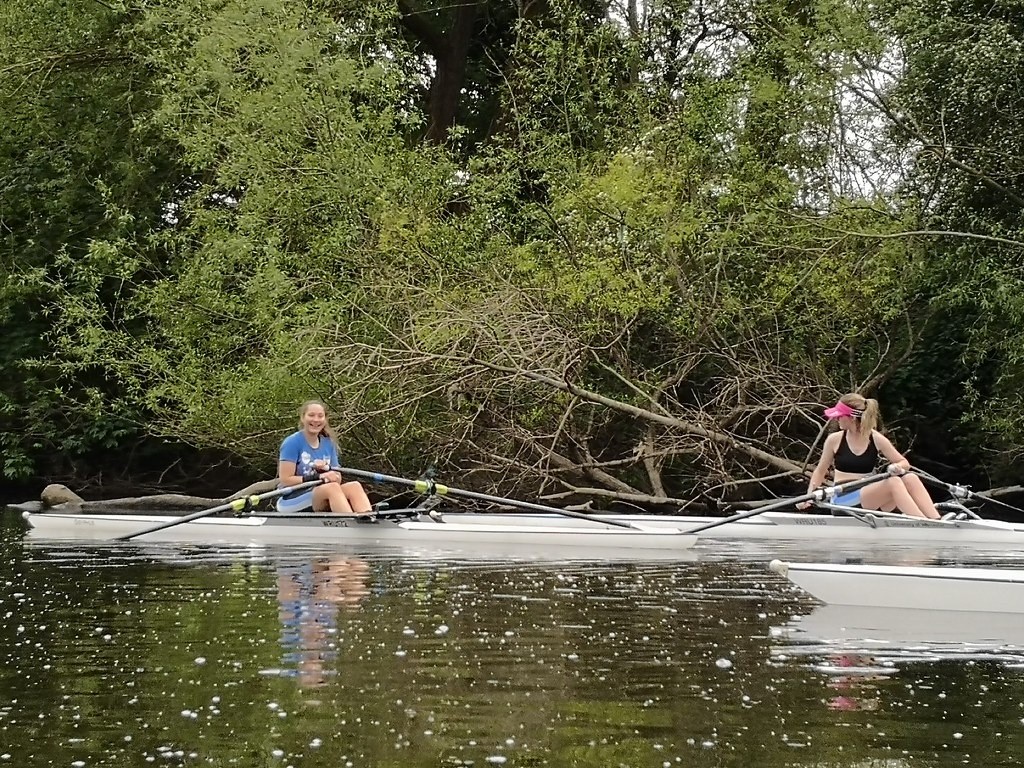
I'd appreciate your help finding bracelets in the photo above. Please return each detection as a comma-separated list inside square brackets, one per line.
[302, 473, 320, 483]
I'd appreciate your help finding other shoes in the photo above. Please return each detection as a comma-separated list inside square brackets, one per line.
[940, 512, 968, 521]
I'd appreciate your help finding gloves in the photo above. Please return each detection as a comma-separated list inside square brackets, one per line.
[795, 500, 814, 510]
[887, 463, 905, 476]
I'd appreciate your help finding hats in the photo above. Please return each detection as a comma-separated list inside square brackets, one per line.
[824, 400, 864, 417]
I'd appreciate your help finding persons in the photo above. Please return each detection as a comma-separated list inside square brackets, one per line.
[795, 393, 967, 520]
[276, 400, 372, 513]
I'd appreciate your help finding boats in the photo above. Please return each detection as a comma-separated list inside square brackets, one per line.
[770, 558, 1024, 612]
[21, 511, 1023, 551]
